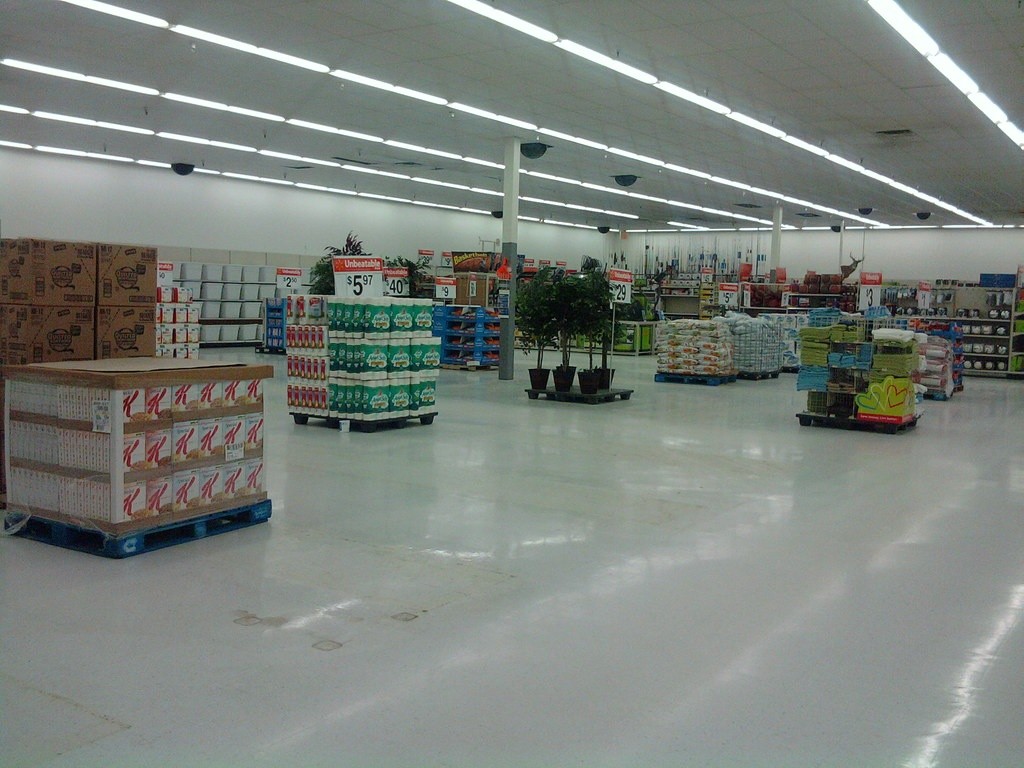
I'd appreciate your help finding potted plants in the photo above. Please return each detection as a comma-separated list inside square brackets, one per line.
[512, 264, 629, 395]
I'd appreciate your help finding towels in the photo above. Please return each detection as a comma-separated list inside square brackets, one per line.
[796, 306, 920, 392]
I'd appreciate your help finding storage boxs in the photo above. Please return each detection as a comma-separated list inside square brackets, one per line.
[0, 237, 312, 367]
[980, 273, 1016, 288]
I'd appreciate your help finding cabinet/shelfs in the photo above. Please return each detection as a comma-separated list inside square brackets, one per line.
[658, 263, 1024, 379]
[171, 278, 278, 344]
